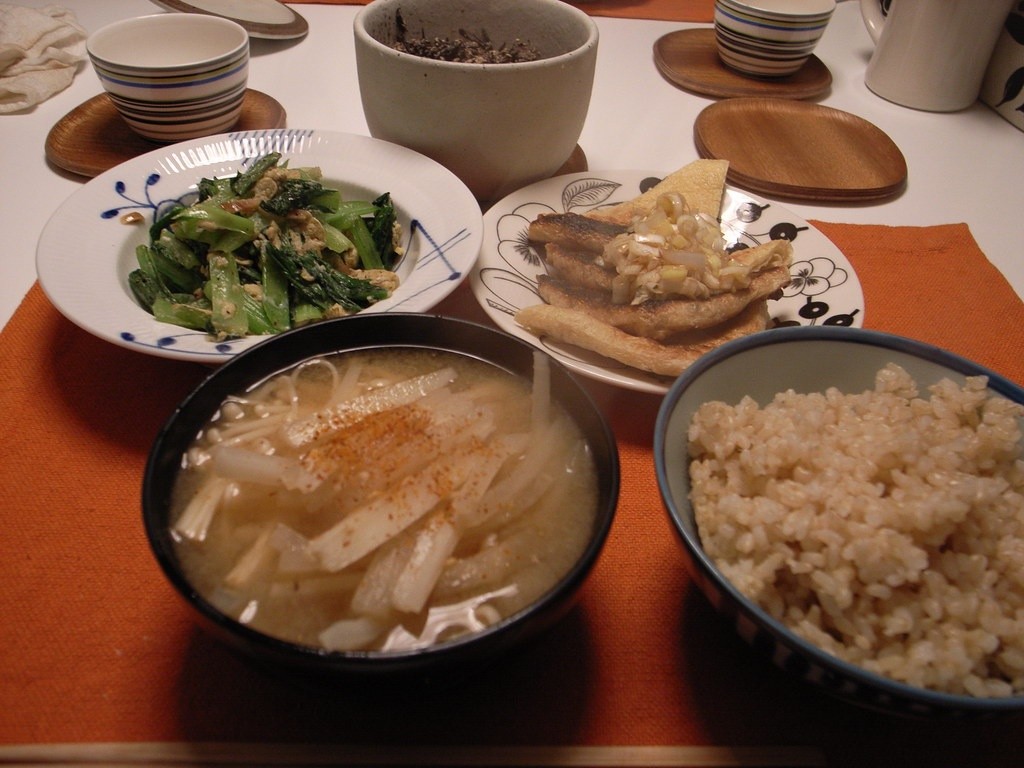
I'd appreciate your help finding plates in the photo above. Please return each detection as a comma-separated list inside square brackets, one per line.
[467, 171, 864, 395]
[36, 129, 483, 364]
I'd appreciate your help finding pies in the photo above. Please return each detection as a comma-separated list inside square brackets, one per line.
[524, 158, 795, 379]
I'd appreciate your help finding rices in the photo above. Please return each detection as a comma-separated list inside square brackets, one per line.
[687, 361, 1024, 697]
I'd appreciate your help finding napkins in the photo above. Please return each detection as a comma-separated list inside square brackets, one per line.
[0, 1, 91, 116]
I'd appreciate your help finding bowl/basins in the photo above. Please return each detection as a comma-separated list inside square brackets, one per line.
[714, 0, 836, 79]
[141, 313, 619, 680]
[85, 13, 249, 146]
[354, 0, 600, 204]
[654, 325, 1024, 724]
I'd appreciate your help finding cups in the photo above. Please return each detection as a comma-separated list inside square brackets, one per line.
[859, 0, 1015, 112]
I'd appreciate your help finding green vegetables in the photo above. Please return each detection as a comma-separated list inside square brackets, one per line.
[127, 152, 397, 340]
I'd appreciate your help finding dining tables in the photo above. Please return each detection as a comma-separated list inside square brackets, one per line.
[1, 1, 1024, 768]
